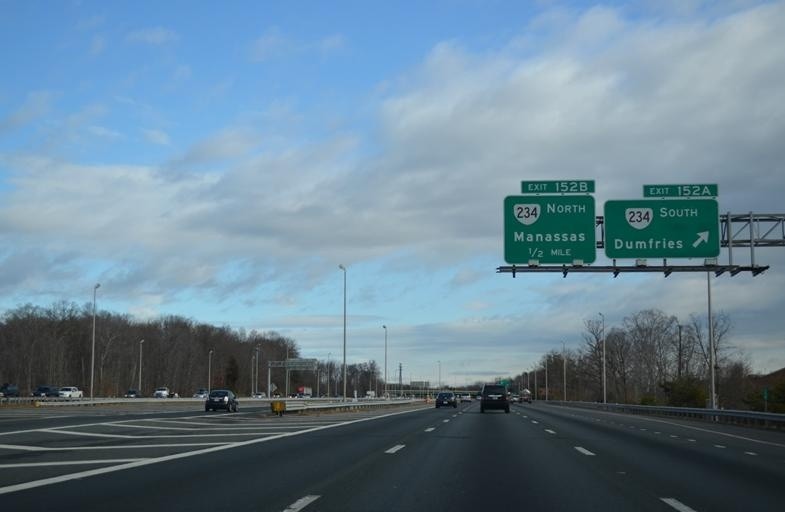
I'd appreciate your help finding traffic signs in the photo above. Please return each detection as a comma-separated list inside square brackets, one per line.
[604, 198, 721, 258]
[504, 196, 595, 263]
[521, 179, 596, 194]
[643, 184, 717, 197]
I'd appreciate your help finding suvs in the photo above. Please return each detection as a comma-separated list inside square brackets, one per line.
[460, 392, 471, 403]
[0, 383, 20, 403]
[480, 383, 511, 414]
[29, 386, 59, 401]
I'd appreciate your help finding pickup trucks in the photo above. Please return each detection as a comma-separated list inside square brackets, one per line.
[57, 386, 83, 398]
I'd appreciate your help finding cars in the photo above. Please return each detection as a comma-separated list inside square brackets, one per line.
[435, 391, 457, 409]
[151, 387, 181, 398]
[476, 394, 481, 401]
[124, 389, 141, 399]
[204, 390, 239, 413]
[192, 389, 206, 399]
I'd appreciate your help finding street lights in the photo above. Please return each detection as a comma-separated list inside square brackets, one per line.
[381, 324, 388, 396]
[338, 263, 348, 397]
[598, 311, 608, 405]
[502, 340, 568, 406]
[248, 345, 443, 400]
[139, 339, 144, 392]
[208, 349, 213, 396]
[90, 282, 102, 399]
